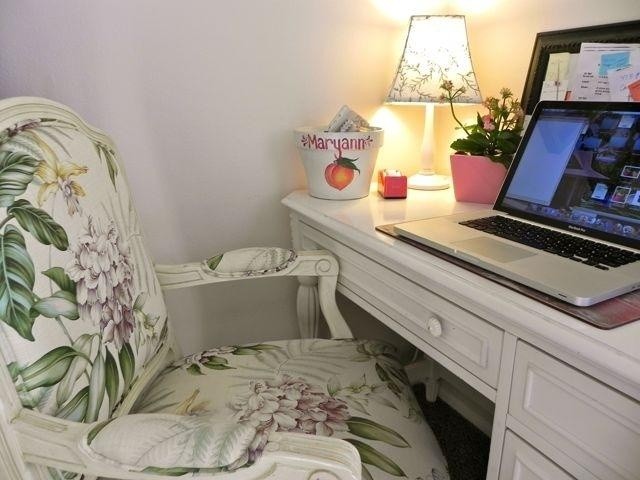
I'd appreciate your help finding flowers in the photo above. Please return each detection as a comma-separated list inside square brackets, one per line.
[439, 80, 525, 169]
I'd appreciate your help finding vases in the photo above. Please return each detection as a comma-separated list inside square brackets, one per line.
[449, 153, 516, 205]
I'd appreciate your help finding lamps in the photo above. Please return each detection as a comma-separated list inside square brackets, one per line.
[385, 16, 482, 189]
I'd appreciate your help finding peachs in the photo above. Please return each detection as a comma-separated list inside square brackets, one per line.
[325, 153, 360, 191]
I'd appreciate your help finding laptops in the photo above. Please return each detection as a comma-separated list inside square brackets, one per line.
[393, 101, 639, 307]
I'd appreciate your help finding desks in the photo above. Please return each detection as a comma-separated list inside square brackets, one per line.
[281, 180, 640, 480]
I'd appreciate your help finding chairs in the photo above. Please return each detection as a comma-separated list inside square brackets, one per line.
[0, 97, 450, 480]
